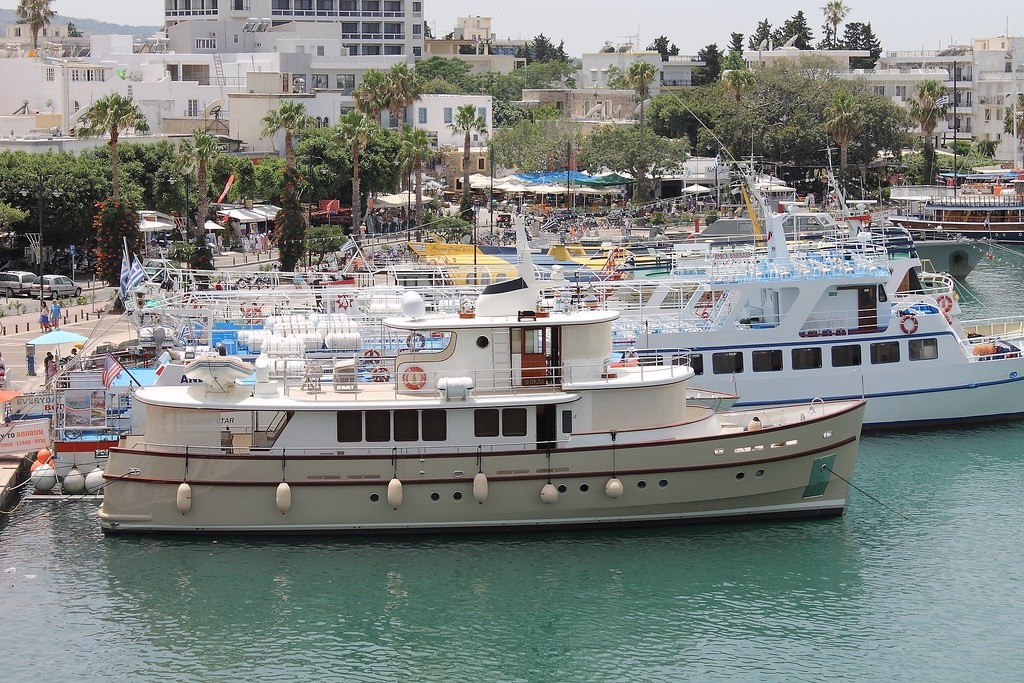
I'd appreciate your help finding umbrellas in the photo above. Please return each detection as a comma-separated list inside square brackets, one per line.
[459, 173, 621, 208]
[681, 183, 712, 212]
[195, 220, 225, 233]
[26, 329, 89, 360]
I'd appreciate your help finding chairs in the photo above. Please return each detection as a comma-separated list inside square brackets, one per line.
[612, 244, 883, 338]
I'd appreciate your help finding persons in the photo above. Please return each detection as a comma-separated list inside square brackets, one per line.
[996, 176, 1002, 185]
[240, 230, 273, 254]
[359, 222, 366, 240]
[491, 197, 498, 214]
[38, 299, 61, 333]
[218, 233, 224, 256]
[460, 229, 473, 244]
[71, 348, 82, 371]
[44, 352, 57, 382]
[374, 210, 446, 233]
[271, 244, 406, 288]
[0, 352, 5, 372]
[607, 192, 709, 218]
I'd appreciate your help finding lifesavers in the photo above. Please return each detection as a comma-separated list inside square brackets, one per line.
[900, 315, 919, 335]
[245, 308, 263, 324]
[937, 295, 952, 312]
[407, 333, 426, 352]
[941, 309, 952, 325]
[364, 349, 381, 364]
[403, 367, 426, 390]
[373, 366, 391, 382]
[183, 296, 199, 311]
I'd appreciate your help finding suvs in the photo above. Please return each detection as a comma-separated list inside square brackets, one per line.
[0, 271, 38, 298]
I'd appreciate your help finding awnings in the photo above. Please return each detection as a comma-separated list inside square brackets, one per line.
[746, 173, 796, 195]
[372, 190, 443, 219]
[135, 218, 174, 259]
[210, 203, 282, 223]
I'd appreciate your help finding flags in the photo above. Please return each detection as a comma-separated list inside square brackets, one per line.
[935, 95, 948, 108]
[102, 351, 122, 388]
[339, 238, 356, 253]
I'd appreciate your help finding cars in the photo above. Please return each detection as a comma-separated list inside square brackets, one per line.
[143, 259, 184, 284]
[30, 275, 83, 300]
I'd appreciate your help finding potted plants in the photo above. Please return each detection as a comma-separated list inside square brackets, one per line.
[222, 221, 231, 251]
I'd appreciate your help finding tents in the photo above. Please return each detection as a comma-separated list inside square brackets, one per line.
[511, 171, 637, 202]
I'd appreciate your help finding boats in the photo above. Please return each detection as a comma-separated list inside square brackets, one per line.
[97, 290, 868, 536]
[0, 67, 1024, 435]
